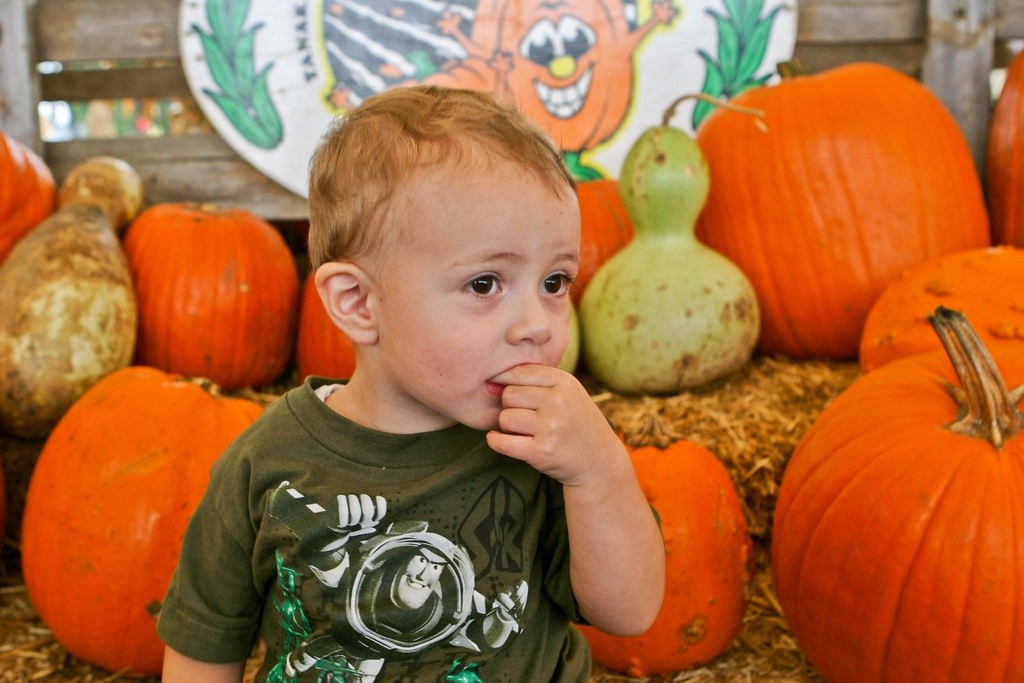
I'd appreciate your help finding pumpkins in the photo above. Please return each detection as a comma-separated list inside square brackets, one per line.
[0, 41, 1024, 683]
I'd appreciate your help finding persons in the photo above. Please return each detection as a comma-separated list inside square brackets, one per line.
[157, 87, 669, 681]
